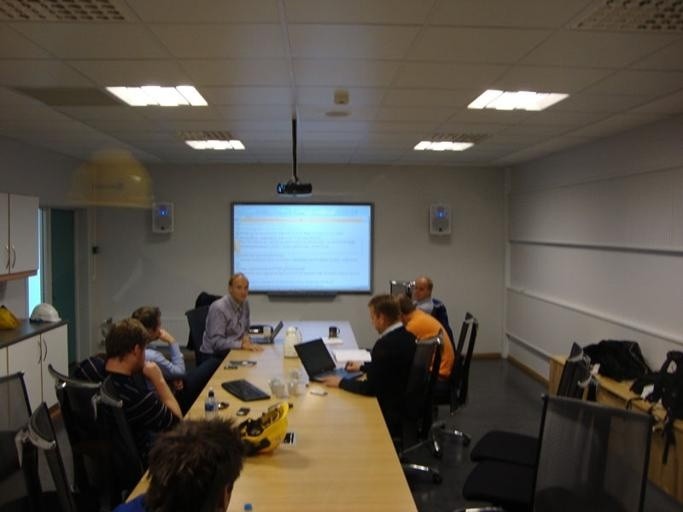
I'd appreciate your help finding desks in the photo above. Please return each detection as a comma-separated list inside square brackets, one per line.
[127, 319, 419, 512]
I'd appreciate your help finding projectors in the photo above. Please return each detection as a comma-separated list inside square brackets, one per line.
[276, 175, 314, 195]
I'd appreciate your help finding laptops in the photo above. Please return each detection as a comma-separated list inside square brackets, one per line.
[222, 379, 270, 401]
[248, 321, 284, 345]
[295, 338, 363, 384]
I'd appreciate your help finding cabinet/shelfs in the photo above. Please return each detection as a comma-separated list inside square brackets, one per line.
[7, 320, 69, 413]
[0, 191, 40, 279]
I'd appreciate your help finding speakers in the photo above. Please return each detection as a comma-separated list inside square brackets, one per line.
[428, 202, 453, 235]
[150, 202, 175, 235]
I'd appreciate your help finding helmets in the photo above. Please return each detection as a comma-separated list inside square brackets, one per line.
[238, 398, 292, 454]
[29, 303, 61, 326]
[0, 304, 18, 333]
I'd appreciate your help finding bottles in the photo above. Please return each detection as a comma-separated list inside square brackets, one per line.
[206, 392, 217, 421]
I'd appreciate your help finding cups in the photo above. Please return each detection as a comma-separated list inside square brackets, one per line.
[329, 326, 340, 338]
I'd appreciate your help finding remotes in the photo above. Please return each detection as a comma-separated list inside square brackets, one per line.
[311, 389, 328, 396]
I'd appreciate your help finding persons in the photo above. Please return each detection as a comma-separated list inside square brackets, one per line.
[111, 415, 247, 512]
[94, 315, 184, 466]
[413, 276, 455, 352]
[319, 294, 429, 441]
[130, 304, 197, 415]
[397, 293, 454, 385]
[198, 272, 264, 364]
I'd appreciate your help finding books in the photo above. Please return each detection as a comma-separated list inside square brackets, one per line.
[332, 348, 372, 363]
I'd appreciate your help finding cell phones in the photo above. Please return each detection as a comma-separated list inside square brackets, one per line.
[236, 408, 249, 416]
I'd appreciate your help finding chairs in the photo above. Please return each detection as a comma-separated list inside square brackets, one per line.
[533, 392, 655, 510]
[50, 365, 105, 456]
[461, 455, 535, 504]
[469, 353, 598, 458]
[430, 310, 491, 443]
[397, 340, 444, 484]
[27, 403, 84, 512]
[184, 308, 216, 360]
[93, 386, 146, 484]
[1, 372, 47, 497]
